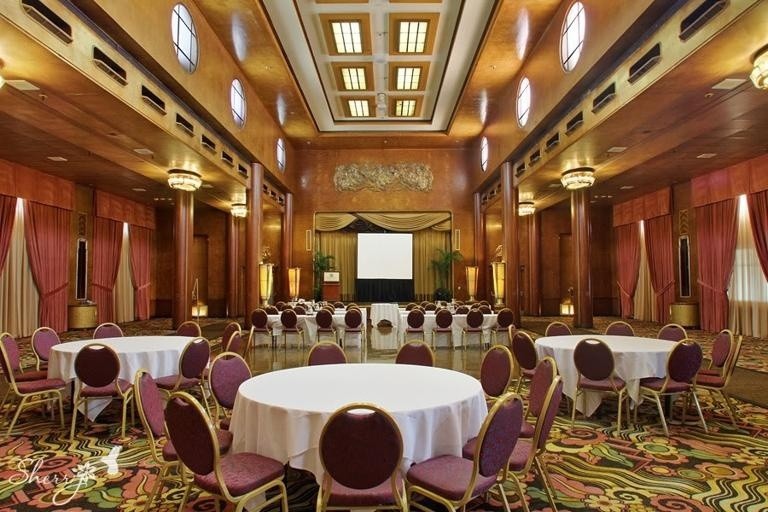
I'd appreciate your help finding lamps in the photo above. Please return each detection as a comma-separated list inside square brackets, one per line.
[461, 262, 479, 303]
[489, 259, 507, 306]
[165, 171, 203, 192]
[558, 165, 598, 192]
[229, 202, 248, 220]
[287, 266, 303, 300]
[748, 44, 768, 94]
[258, 260, 275, 305]
[514, 200, 538, 220]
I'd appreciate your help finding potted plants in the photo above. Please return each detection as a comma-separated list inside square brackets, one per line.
[427, 244, 465, 304]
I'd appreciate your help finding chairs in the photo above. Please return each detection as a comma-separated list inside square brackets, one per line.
[0, 295, 752, 512]
